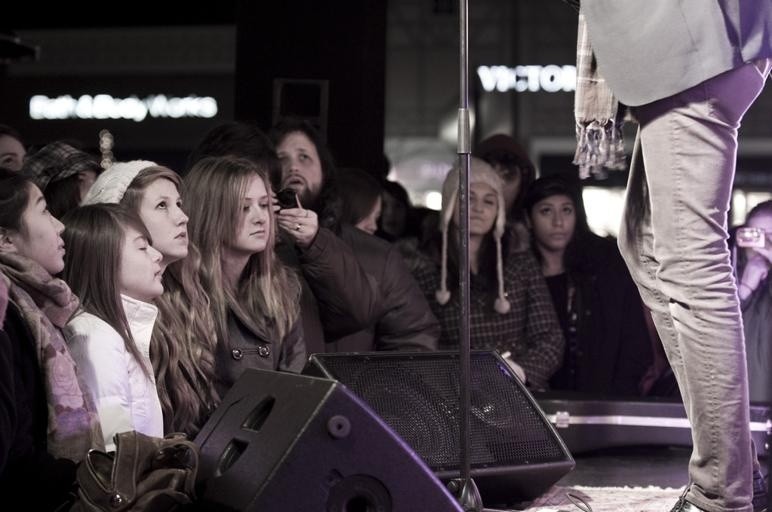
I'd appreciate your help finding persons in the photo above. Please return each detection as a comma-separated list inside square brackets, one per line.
[269, 113, 442, 352]
[326, 170, 383, 236]
[182, 155, 308, 402]
[473, 133, 535, 223]
[517, 176, 658, 399]
[184, 121, 379, 356]
[80, 160, 221, 438]
[0, 173, 106, 511]
[56, 201, 166, 452]
[380, 180, 411, 237]
[736, 198, 772, 406]
[25, 140, 104, 223]
[574, 2, 772, 511]
[0, 133, 27, 172]
[406, 156, 567, 393]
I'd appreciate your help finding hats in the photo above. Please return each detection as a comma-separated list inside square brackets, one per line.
[82, 160, 158, 205]
[21, 140, 105, 191]
[439, 155, 505, 238]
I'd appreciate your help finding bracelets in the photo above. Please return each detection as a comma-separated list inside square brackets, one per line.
[740, 281, 754, 293]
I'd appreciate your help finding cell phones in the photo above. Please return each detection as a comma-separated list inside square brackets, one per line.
[276, 191, 303, 209]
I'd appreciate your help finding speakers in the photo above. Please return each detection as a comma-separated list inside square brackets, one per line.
[189, 365, 462, 510]
[300, 348, 578, 512]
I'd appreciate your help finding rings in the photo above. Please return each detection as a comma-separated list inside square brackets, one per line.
[294, 225, 300, 230]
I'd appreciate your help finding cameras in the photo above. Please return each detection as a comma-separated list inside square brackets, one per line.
[736, 227, 766, 248]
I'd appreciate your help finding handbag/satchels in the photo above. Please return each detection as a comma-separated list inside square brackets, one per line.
[73, 431, 199, 511]
